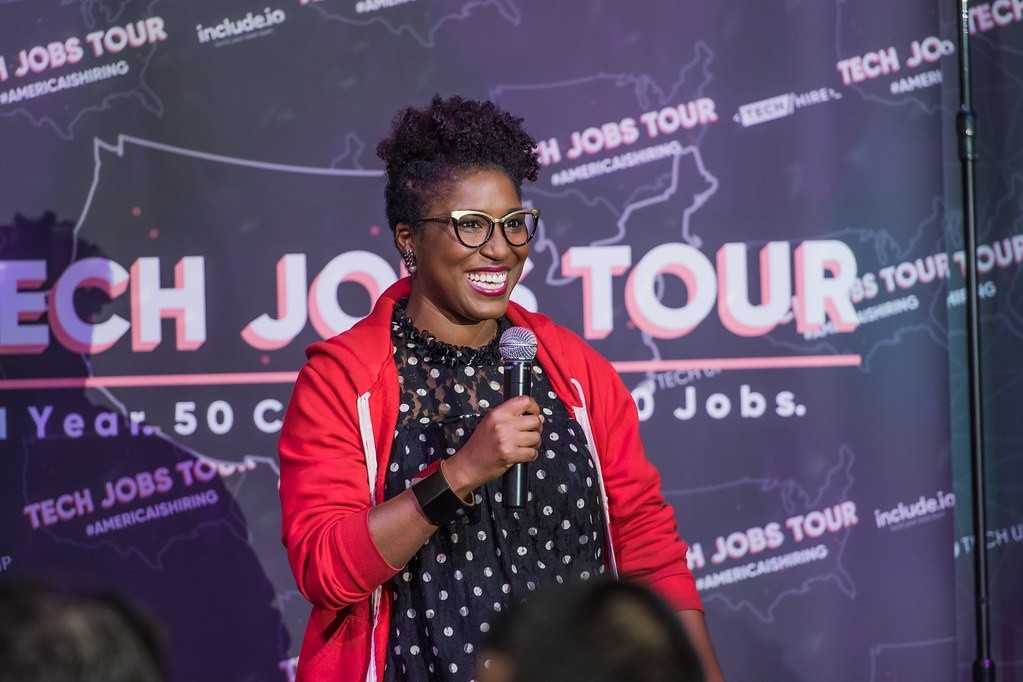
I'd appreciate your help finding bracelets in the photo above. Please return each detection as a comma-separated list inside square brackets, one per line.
[414, 460, 481, 526]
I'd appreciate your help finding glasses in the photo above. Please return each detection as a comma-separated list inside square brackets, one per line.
[410, 208, 540, 248]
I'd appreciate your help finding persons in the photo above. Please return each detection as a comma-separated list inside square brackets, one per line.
[469, 574, 704, 682]
[0, 588, 167, 681]
[280, 98, 720, 682]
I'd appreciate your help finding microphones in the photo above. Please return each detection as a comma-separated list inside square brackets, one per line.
[497, 327, 537, 509]
